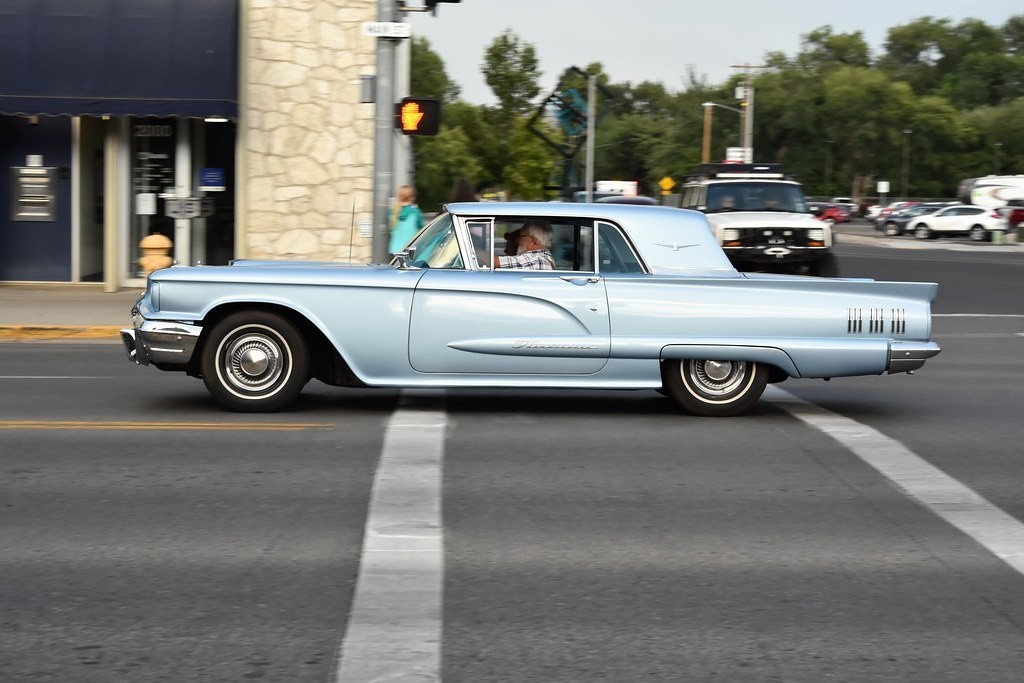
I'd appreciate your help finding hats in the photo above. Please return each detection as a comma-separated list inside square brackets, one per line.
[504, 228, 521, 239]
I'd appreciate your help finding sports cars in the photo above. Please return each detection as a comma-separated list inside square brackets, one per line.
[119, 200, 941, 419]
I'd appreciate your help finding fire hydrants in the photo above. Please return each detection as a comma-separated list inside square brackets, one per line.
[138, 232, 178, 278]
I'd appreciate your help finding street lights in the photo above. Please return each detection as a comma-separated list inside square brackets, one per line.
[702, 102, 753, 164]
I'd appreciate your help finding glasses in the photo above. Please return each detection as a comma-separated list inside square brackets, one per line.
[517, 234, 533, 239]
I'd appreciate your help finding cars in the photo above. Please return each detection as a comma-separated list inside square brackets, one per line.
[905, 205, 1010, 243]
[802, 173, 1024, 237]
[566, 186, 671, 269]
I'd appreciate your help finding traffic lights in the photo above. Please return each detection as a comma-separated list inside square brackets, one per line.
[401, 96, 440, 136]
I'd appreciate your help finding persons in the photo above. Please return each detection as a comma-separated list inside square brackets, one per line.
[718, 194, 737, 212]
[387, 186, 425, 266]
[473, 221, 558, 271]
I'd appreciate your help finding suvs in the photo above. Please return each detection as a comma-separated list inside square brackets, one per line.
[674, 162, 834, 277]
